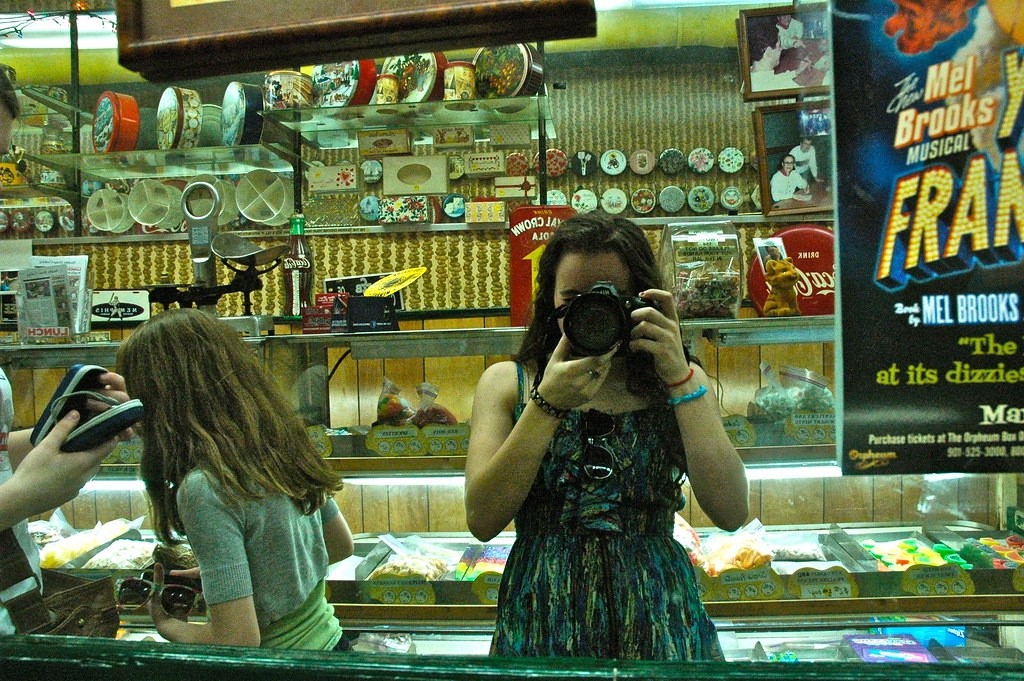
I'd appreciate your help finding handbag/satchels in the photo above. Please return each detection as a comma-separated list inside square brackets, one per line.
[15, 568, 120, 638]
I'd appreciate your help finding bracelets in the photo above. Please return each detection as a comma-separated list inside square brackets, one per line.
[664, 368, 695, 388]
[529, 386, 570, 420]
[667, 384, 707, 406]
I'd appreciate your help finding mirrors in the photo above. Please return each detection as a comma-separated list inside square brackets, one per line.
[0, 0, 1024, 681]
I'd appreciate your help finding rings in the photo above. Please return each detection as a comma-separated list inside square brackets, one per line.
[588, 370, 601, 379]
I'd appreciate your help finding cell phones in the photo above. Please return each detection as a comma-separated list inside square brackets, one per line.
[140, 572, 203, 594]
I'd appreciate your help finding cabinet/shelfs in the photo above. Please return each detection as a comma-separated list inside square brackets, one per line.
[0, 313, 1024, 635]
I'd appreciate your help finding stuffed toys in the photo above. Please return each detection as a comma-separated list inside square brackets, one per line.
[763, 256, 802, 316]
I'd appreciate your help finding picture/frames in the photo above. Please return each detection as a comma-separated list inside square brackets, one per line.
[754, 101, 833, 218]
[739, 3, 833, 102]
[115, 0, 595, 73]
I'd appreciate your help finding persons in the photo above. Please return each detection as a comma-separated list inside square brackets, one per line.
[0, 63, 134, 634]
[764, 246, 780, 265]
[465, 211, 749, 662]
[775, 14, 803, 49]
[116, 308, 355, 653]
[747, 23, 829, 92]
[788, 136, 824, 184]
[770, 154, 810, 202]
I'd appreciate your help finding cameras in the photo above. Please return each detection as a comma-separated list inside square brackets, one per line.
[563, 280, 660, 357]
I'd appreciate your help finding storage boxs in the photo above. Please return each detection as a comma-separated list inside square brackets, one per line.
[432, 125, 476, 151]
[489, 124, 531, 150]
[308, 166, 361, 193]
[358, 127, 412, 160]
[382, 154, 451, 196]
[379, 195, 433, 225]
[494, 175, 538, 200]
[465, 201, 508, 224]
[463, 151, 508, 179]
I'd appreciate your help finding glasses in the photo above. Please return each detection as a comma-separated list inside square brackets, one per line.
[580, 408, 615, 479]
[783, 162, 794, 165]
[117, 578, 202, 620]
